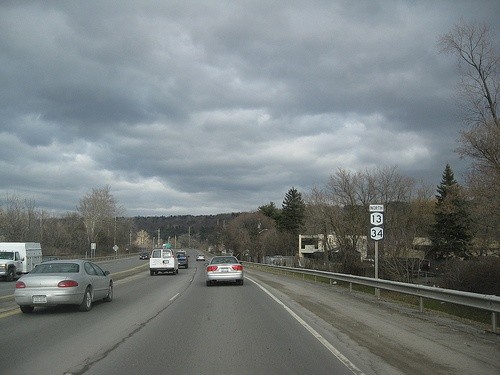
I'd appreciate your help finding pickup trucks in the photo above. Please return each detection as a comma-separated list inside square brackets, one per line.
[176, 253, 189, 267]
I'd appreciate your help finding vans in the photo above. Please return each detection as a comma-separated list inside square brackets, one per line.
[149, 246, 179, 274]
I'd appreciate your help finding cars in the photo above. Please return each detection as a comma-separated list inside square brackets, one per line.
[196, 255, 205, 261]
[205, 255, 244, 285]
[14, 258, 115, 311]
[139, 251, 149, 260]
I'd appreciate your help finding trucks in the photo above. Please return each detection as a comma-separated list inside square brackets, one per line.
[1, 241, 45, 280]
